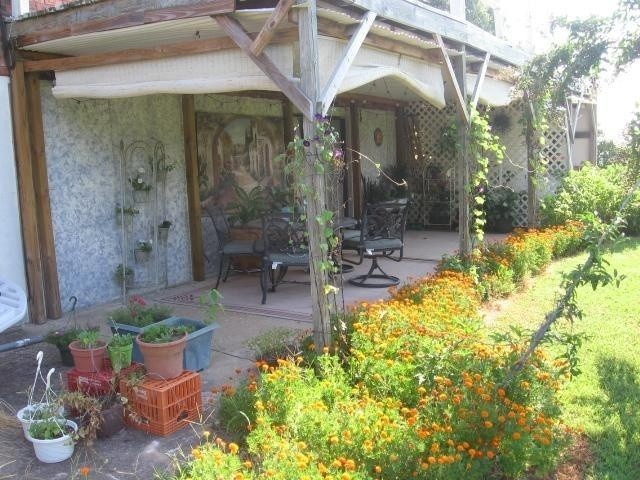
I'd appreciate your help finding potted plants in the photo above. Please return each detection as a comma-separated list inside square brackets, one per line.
[18, 285, 228, 463]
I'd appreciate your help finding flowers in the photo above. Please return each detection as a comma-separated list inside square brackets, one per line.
[296, 108, 345, 165]
[113, 182, 171, 285]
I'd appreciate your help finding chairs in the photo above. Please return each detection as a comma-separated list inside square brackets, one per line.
[201, 194, 408, 305]
